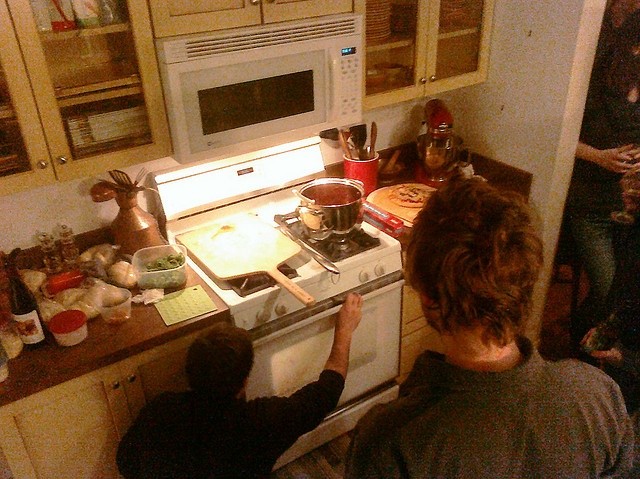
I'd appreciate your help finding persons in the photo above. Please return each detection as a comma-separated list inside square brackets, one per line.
[346, 173, 634, 478]
[579, 299, 640, 376]
[115, 290, 365, 479]
[565, 1, 639, 336]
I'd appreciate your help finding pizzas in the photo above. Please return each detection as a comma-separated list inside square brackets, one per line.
[390, 185, 428, 208]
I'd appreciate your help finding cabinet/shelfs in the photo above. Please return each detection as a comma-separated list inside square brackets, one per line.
[364, 0, 499, 113]
[0, 0, 173, 197]
[148, 0, 354, 39]
[397, 283, 446, 387]
[0, 322, 233, 479]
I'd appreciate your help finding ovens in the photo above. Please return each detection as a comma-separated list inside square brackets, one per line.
[233, 280, 412, 472]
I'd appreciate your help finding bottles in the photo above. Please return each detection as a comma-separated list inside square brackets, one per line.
[40, 235, 64, 274]
[6, 268, 48, 350]
[56, 224, 79, 267]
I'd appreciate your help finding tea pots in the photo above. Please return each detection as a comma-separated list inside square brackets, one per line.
[417, 99, 472, 189]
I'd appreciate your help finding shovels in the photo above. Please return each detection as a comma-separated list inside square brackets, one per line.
[108, 169, 134, 192]
[369, 121, 378, 161]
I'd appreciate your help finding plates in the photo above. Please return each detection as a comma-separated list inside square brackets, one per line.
[363, 1, 393, 45]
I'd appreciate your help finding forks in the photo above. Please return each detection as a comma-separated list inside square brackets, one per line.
[134, 166, 149, 189]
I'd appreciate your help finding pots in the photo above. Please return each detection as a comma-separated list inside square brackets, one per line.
[292, 176, 365, 232]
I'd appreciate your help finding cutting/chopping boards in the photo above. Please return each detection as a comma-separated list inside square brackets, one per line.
[176, 212, 317, 307]
[366, 182, 440, 227]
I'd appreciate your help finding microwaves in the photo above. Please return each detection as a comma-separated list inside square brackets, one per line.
[159, 13, 364, 166]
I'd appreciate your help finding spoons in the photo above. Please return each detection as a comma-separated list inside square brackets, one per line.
[89, 182, 125, 203]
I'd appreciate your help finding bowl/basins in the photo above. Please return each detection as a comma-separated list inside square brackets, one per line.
[377, 158, 405, 179]
[94, 289, 133, 325]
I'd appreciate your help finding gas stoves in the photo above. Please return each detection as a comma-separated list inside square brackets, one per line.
[166, 194, 402, 331]
